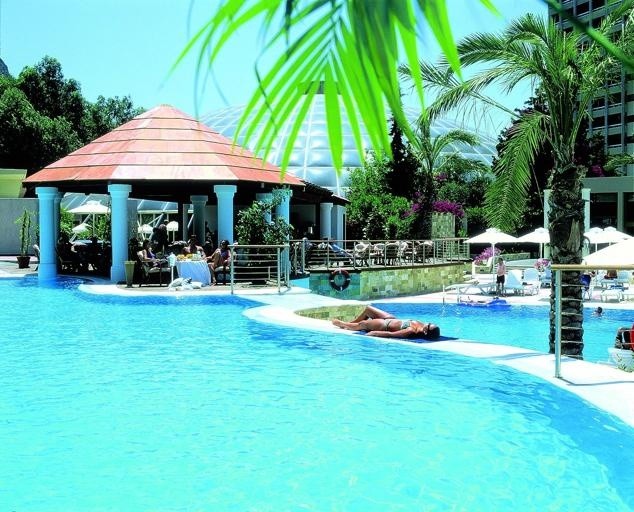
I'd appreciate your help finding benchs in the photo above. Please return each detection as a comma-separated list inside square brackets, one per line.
[289, 239, 354, 268]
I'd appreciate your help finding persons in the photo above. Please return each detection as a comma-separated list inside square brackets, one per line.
[141, 217, 233, 288]
[591, 306, 604, 316]
[494, 256, 507, 296]
[85, 235, 103, 264]
[332, 304, 440, 342]
[457, 295, 500, 306]
[57, 238, 82, 274]
[602, 269, 617, 280]
[288, 235, 358, 266]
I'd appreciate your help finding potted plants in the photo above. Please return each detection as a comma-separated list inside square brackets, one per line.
[12, 206, 39, 269]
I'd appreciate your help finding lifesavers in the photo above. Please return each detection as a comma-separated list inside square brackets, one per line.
[330, 270, 351, 290]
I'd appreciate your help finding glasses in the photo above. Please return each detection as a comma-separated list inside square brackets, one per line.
[428, 323, 431, 332]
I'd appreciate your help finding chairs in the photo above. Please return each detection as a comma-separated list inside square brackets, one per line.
[53, 239, 238, 288]
[354, 237, 435, 267]
[500, 267, 542, 297]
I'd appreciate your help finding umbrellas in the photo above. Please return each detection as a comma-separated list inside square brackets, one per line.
[582, 227, 619, 251]
[460, 228, 518, 275]
[514, 225, 552, 260]
[603, 225, 632, 246]
